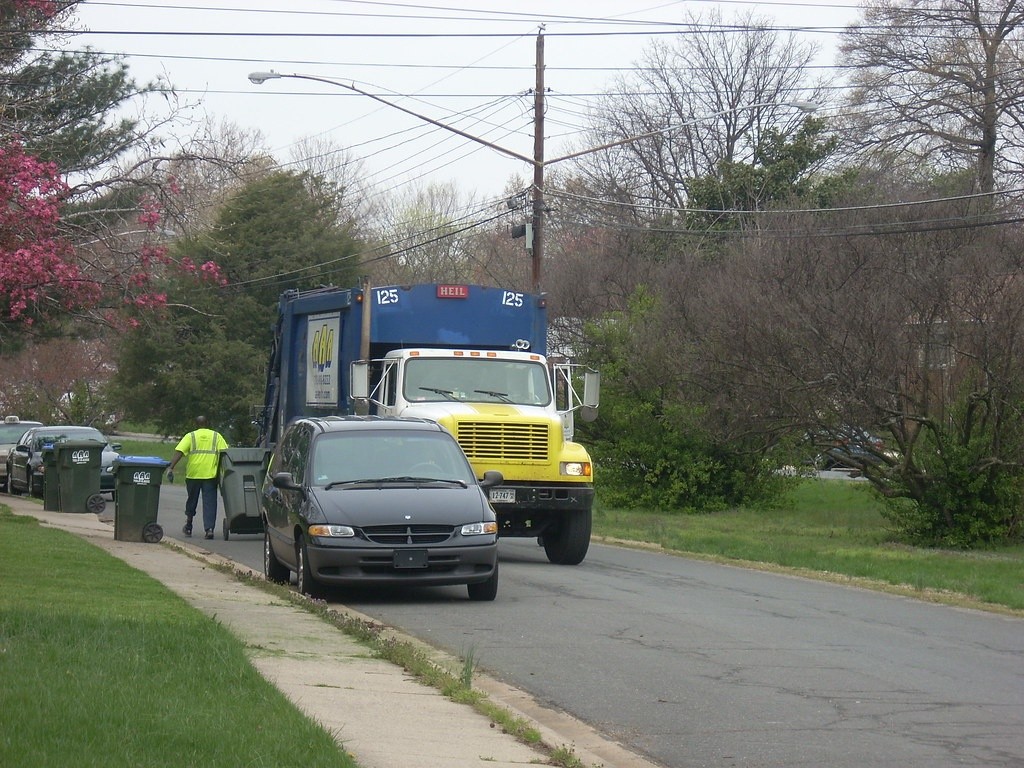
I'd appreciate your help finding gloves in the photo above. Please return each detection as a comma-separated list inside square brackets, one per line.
[167, 468, 175, 484]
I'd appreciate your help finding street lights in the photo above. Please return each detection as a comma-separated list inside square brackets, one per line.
[247, 71, 544, 297]
[532, 100, 817, 297]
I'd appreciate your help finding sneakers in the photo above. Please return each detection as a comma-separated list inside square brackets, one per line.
[183, 524, 192, 537]
[205, 528, 214, 539]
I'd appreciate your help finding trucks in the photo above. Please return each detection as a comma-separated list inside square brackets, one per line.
[264, 284, 601, 564]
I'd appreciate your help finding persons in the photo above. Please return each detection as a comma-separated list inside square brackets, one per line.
[167, 416, 228, 539]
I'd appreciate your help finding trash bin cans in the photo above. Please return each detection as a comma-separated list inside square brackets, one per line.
[216, 446, 274, 539]
[40, 440, 61, 510]
[54, 436, 108, 514]
[110, 455, 172, 543]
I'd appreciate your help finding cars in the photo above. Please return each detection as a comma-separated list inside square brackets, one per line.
[259, 412, 498, 601]
[7, 426, 122, 501]
[0, 414, 42, 487]
